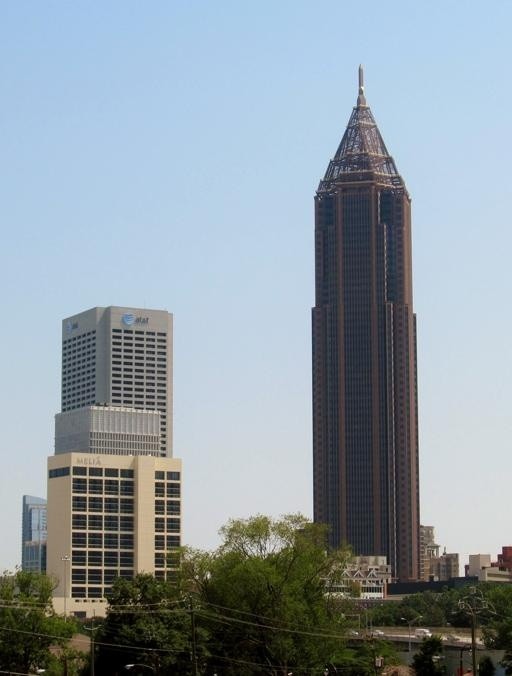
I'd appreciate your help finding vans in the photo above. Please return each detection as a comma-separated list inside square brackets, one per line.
[124, 663, 158, 676]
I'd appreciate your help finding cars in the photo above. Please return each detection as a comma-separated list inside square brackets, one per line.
[351, 630, 384, 637]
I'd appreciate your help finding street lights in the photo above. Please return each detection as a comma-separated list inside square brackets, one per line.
[400, 616, 424, 663]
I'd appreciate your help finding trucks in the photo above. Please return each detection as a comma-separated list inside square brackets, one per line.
[414, 629, 432, 639]
